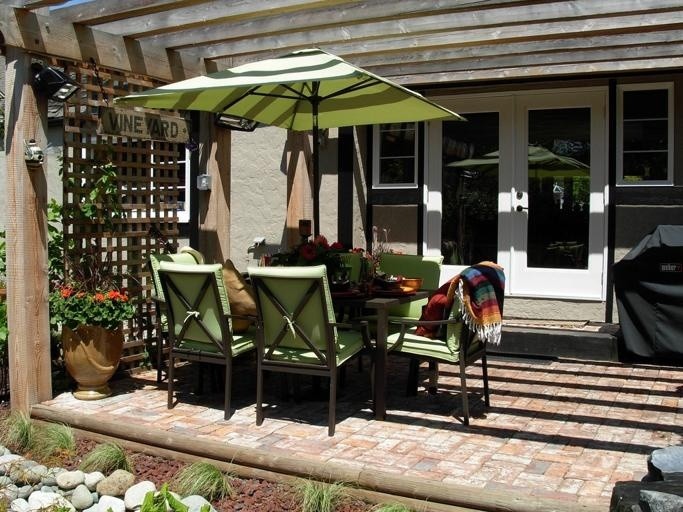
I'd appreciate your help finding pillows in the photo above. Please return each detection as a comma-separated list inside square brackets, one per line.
[445, 295, 480, 352]
[221, 258, 259, 336]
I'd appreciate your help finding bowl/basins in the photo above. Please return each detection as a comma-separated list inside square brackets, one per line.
[401, 277, 424, 292]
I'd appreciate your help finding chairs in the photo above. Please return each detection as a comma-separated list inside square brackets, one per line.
[157, 257, 258, 421]
[247, 264, 370, 436]
[359, 250, 441, 343]
[148, 245, 205, 383]
[361, 260, 505, 425]
[333, 251, 365, 287]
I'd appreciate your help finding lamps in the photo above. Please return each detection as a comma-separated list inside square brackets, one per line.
[29, 62, 81, 103]
[214, 113, 259, 132]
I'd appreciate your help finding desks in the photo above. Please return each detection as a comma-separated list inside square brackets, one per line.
[244, 274, 438, 422]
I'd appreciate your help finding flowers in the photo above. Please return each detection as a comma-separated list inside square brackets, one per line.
[48, 280, 138, 333]
[269, 232, 343, 266]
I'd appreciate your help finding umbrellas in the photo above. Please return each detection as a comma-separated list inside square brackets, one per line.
[446, 139, 594, 176]
[115, 49, 467, 241]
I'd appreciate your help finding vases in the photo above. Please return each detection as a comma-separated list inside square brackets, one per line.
[62, 319, 123, 401]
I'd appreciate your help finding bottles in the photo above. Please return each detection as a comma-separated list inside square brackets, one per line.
[359, 258, 367, 282]
